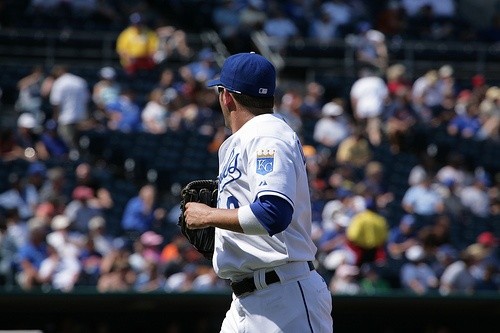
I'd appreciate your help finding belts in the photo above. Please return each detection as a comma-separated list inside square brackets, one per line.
[231, 260, 315, 298]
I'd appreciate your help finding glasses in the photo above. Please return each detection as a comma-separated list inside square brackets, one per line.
[216, 84, 242, 94]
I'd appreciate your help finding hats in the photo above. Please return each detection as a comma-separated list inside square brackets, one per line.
[74, 187, 92, 198]
[140, 231, 162, 245]
[101, 67, 116, 78]
[321, 101, 343, 116]
[206, 52, 276, 96]
[17, 113, 35, 127]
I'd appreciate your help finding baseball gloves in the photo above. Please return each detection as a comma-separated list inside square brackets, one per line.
[175, 179, 219, 260]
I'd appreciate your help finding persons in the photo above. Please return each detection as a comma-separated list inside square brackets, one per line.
[178, 49, 334, 333]
[0, 0, 500, 297]
